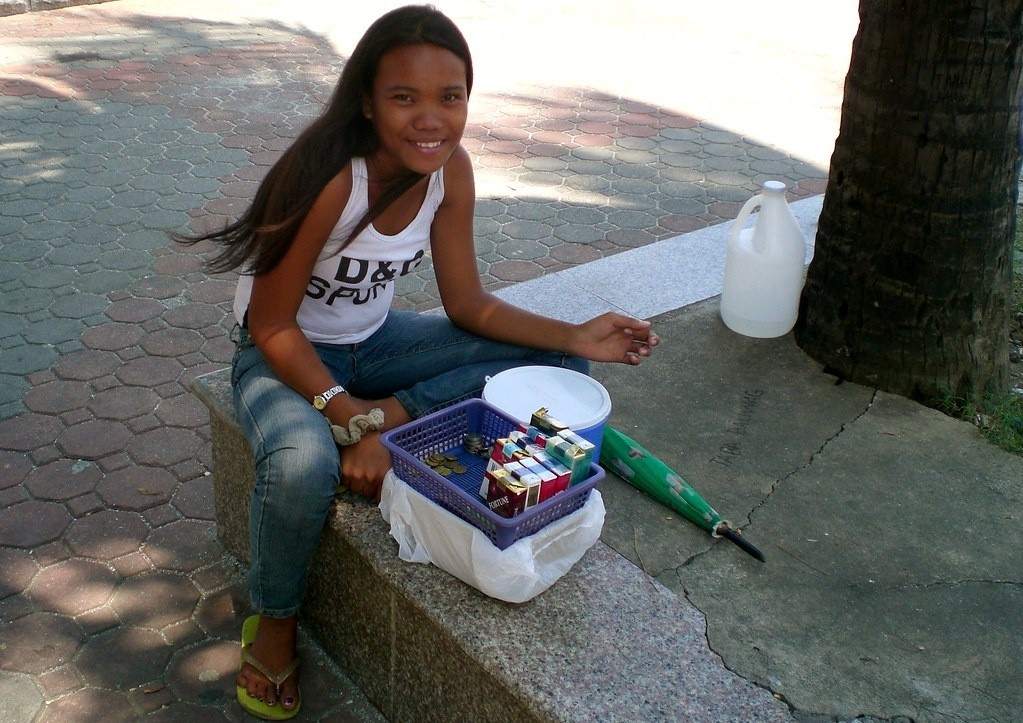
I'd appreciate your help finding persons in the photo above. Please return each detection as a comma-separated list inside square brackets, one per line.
[162, 5, 661, 720]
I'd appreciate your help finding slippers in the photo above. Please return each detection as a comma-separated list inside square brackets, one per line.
[237, 614, 302, 720]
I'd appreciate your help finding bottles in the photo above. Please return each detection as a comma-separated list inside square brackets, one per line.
[720, 181, 808, 339]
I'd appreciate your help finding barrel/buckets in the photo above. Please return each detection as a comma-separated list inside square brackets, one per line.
[482, 367, 612, 483]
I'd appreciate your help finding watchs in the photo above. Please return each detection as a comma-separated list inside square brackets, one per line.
[312, 385, 349, 411]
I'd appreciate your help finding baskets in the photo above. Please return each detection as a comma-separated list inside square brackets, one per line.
[379, 397, 608, 551]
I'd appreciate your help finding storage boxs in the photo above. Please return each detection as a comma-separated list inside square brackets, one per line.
[380, 398, 607, 551]
